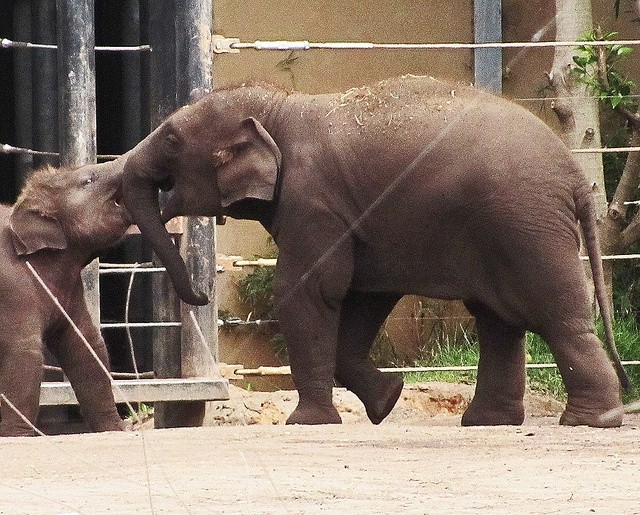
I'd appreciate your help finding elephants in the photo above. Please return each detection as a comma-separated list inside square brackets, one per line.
[0, 160, 136, 437]
[119, 74, 631, 428]
